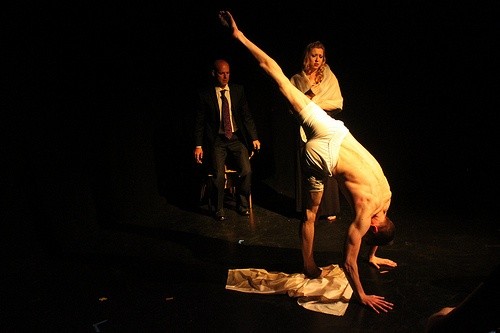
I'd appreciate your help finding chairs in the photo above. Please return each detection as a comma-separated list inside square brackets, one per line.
[199, 151, 255, 217]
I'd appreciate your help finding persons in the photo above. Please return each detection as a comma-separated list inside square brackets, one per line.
[218, 10, 398, 314]
[194, 60, 261, 222]
[287, 42, 343, 222]
